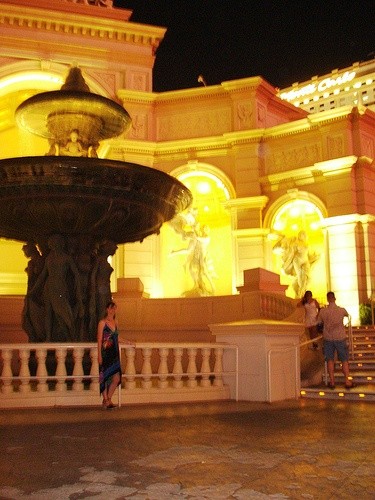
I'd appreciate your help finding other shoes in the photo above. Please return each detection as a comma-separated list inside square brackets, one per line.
[108, 398, 117, 408]
[103, 399, 115, 410]
[314, 345, 319, 351]
[328, 383, 335, 389]
[346, 383, 357, 388]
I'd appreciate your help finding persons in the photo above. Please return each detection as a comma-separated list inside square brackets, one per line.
[318, 291, 356, 388]
[174, 211, 216, 298]
[297, 290, 322, 351]
[56, 128, 93, 157]
[275, 230, 320, 297]
[97, 301, 121, 409]
